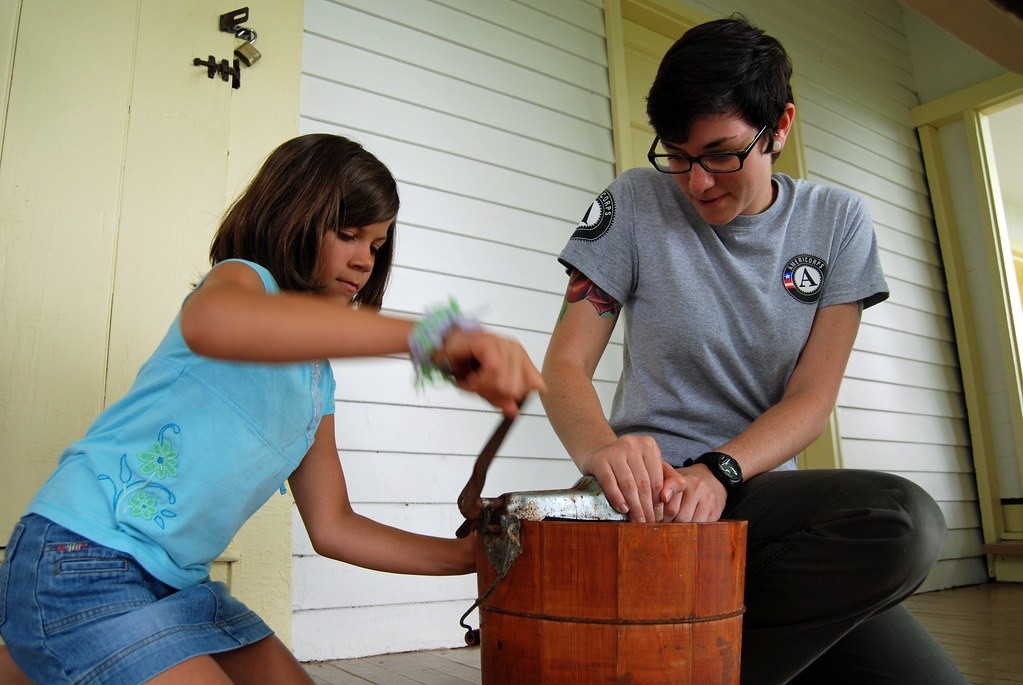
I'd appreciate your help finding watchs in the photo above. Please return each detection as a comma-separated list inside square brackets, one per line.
[683, 451, 744, 495]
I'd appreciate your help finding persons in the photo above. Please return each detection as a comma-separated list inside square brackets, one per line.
[539, 12, 970, 685]
[0, 133, 548, 685]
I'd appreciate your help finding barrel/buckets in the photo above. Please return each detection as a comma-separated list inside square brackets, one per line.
[472, 515, 748, 683]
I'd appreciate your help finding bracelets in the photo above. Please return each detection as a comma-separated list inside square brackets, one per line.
[408, 296, 481, 396]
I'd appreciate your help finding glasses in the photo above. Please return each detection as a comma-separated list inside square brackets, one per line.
[648, 125, 770, 173]
